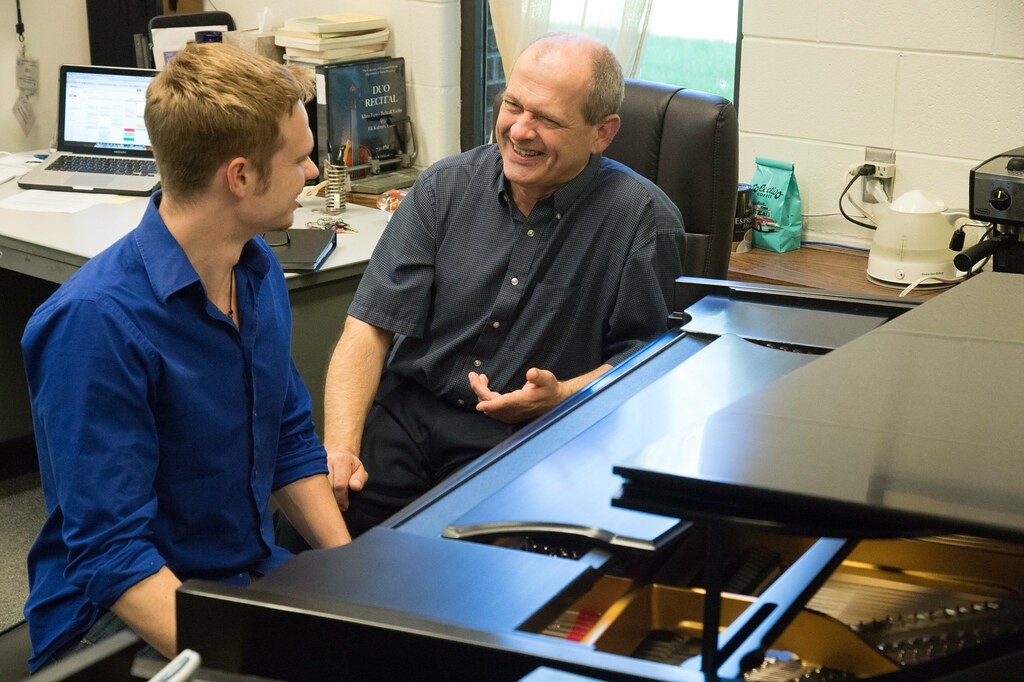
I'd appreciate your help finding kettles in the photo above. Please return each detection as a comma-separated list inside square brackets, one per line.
[868, 190, 992, 284]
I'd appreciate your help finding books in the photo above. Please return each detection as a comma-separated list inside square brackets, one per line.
[274, 12, 425, 211]
[256, 228, 337, 273]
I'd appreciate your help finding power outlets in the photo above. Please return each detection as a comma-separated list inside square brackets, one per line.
[862, 146, 896, 204]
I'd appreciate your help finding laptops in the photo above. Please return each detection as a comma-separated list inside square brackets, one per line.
[17, 63, 161, 196]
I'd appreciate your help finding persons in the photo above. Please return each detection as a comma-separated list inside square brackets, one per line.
[18, 43, 356, 674]
[273, 34, 689, 551]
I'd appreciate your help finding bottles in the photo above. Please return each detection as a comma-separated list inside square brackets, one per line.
[49, 136, 57, 156]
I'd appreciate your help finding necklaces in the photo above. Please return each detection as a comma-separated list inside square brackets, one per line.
[223, 267, 238, 321]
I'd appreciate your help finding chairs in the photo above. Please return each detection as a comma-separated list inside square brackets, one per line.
[603, 78, 738, 313]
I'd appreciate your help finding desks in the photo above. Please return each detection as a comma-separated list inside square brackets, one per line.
[0, 147, 393, 443]
[727, 242, 949, 300]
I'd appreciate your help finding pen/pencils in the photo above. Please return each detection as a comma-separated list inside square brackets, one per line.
[327, 139, 352, 207]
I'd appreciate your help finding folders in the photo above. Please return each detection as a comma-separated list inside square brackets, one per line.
[255, 228, 337, 274]
